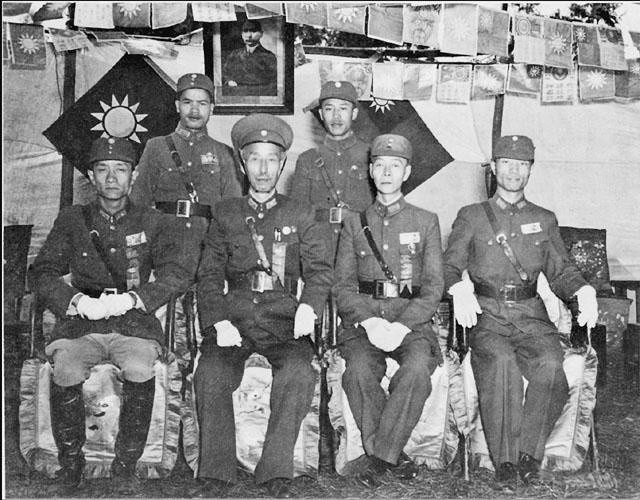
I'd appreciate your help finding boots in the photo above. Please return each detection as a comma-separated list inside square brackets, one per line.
[39, 380, 89, 496]
[108, 377, 156, 496]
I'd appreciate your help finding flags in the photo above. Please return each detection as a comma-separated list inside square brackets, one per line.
[38, 50, 182, 189]
[301, 95, 457, 203]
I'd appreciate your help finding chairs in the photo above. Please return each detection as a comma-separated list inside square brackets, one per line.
[3, 220, 37, 362]
[550, 224, 639, 380]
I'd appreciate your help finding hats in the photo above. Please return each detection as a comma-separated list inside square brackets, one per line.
[242, 19, 262, 31]
[319, 79, 359, 104]
[88, 134, 136, 163]
[230, 112, 294, 149]
[492, 134, 537, 161]
[175, 72, 216, 95]
[370, 133, 414, 160]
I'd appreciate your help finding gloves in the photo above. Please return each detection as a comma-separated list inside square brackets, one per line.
[363, 317, 411, 352]
[99, 290, 134, 318]
[214, 321, 244, 349]
[449, 282, 483, 329]
[294, 305, 317, 340]
[74, 294, 109, 321]
[577, 284, 601, 330]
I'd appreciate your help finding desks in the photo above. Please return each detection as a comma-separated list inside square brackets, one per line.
[608, 254, 639, 325]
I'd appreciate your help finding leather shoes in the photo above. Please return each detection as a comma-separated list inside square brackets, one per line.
[266, 477, 301, 499]
[185, 476, 230, 499]
[493, 461, 519, 494]
[390, 450, 421, 481]
[357, 455, 390, 488]
[519, 458, 547, 489]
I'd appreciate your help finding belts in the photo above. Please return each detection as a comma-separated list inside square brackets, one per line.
[154, 199, 213, 219]
[358, 279, 422, 302]
[72, 284, 138, 299]
[473, 284, 539, 298]
[229, 271, 293, 293]
[313, 207, 365, 224]
[234, 81, 272, 87]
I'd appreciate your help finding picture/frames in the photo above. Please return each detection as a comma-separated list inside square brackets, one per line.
[202, 17, 295, 116]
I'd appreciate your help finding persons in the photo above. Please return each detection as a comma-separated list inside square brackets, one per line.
[196, 110, 336, 498]
[287, 79, 375, 256]
[331, 134, 449, 492]
[442, 132, 599, 499]
[219, 17, 280, 97]
[28, 136, 204, 497]
[129, 70, 246, 486]
